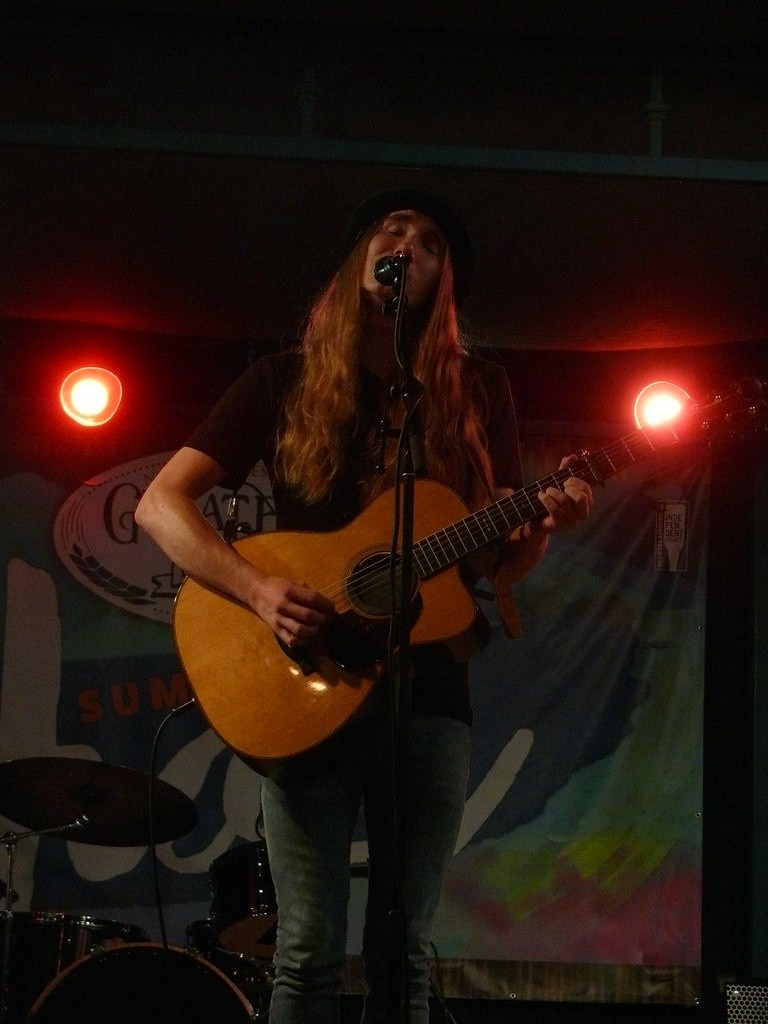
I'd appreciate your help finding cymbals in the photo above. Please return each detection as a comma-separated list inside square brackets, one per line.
[1, 754, 199, 849]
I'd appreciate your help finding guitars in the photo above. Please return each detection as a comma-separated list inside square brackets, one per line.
[172, 375, 768, 770]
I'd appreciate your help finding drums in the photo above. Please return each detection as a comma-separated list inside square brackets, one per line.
[205, 838, 280, 957]
[27, 942, 262, 1024]
[0, 908, 150, 1024]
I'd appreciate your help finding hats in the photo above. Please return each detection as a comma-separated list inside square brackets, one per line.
[342, 187, 475, 313]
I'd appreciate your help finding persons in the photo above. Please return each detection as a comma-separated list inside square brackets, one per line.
[134, 197, 594, 1024]
